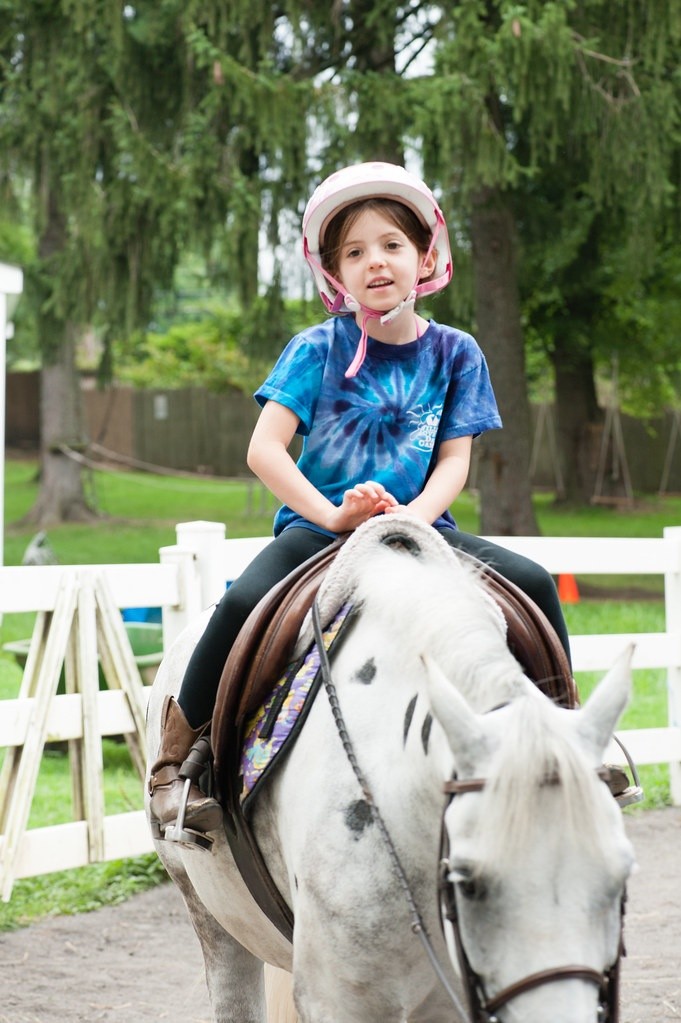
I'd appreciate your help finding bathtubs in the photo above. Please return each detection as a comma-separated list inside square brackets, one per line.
[4, 622, 164, 695]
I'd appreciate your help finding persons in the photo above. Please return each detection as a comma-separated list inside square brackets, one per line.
[147, 161, 631, 838]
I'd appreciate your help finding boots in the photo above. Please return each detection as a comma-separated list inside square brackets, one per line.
[146, 693, 222, 840]
[570, 678, 628, 796]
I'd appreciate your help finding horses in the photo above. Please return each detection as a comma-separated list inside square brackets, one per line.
[141, 509, 639, 1023]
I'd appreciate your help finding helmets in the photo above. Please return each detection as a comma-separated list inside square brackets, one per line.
[300, 160, 454, 311]
